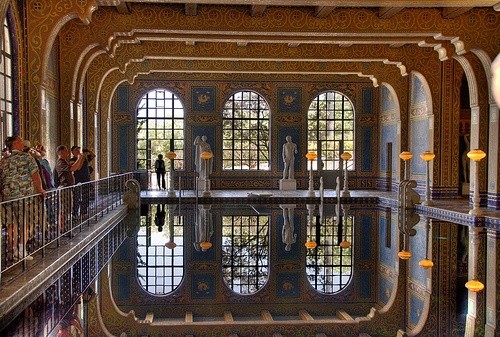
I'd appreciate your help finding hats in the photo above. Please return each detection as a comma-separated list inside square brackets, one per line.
[24, 140, 31, 147]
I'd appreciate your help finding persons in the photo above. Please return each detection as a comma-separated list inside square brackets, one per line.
[69, 147, 90, 219]
[36, 253, 97, 337]
[23, 140, 47, 250]
[282, 208, 297, 251]
[0, 136, 45, 261]
[194, 135, 213, 178]
[155, 154, 166, 190]
[199, 210, 213, 251]
[35, 145, 57, 231]
[154, 203, 166, 231]
[83, 149, 90, 163]
[282, 136, 298, 180]
[53, 145, 85, 237]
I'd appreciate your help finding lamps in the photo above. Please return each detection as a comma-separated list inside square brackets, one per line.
[305, 204, 316, 249]
[340, 204, 350, 247]
[166, 152, 176, 197]
[465, 227, 484, 292]
[398, 234, 412, 260]
[467, 149, 486, 215]
[166, 204, 176, 248]
[306, 152, 317, 196]
[420, 151, 435, 206]
[200, 152, 212, 197]
[200, 204, 212, 249]
[340, 152, 352, 195]
[419, 217, 434, 269]
[399, 150, 413, 180]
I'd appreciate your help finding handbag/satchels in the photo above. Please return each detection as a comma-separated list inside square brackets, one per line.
[52, 168, 60, 188]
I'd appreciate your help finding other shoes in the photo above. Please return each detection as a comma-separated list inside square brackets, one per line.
[13, 250, 33, 260]
